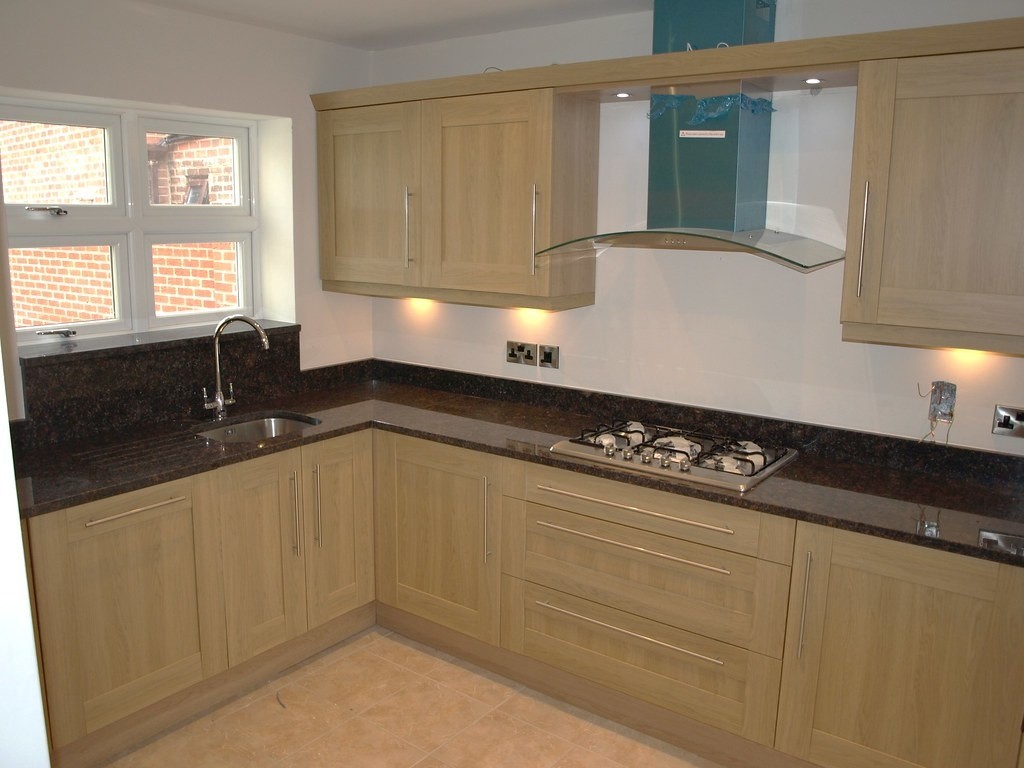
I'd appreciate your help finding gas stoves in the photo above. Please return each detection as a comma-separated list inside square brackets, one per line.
[547, 420, 800, 495]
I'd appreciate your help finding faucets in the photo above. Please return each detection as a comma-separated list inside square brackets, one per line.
[213, 314, 271, 382]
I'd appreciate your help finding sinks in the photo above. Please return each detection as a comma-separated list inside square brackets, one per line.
[190, 409, 322, 444]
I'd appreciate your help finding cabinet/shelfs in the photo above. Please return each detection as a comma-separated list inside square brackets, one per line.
[373, 429, 502, 671]
[27, 467, 229, 768]
[839, 47, 1024, 354]
[501, 454, 797, 768]
[217, 427, 373, 707]
[423, 88, 601, 311]
[317, 99, 423, 298]
[774, 519, 1024, 768]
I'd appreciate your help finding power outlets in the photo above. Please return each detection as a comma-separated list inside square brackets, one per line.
[507, 340, 537, 366]
